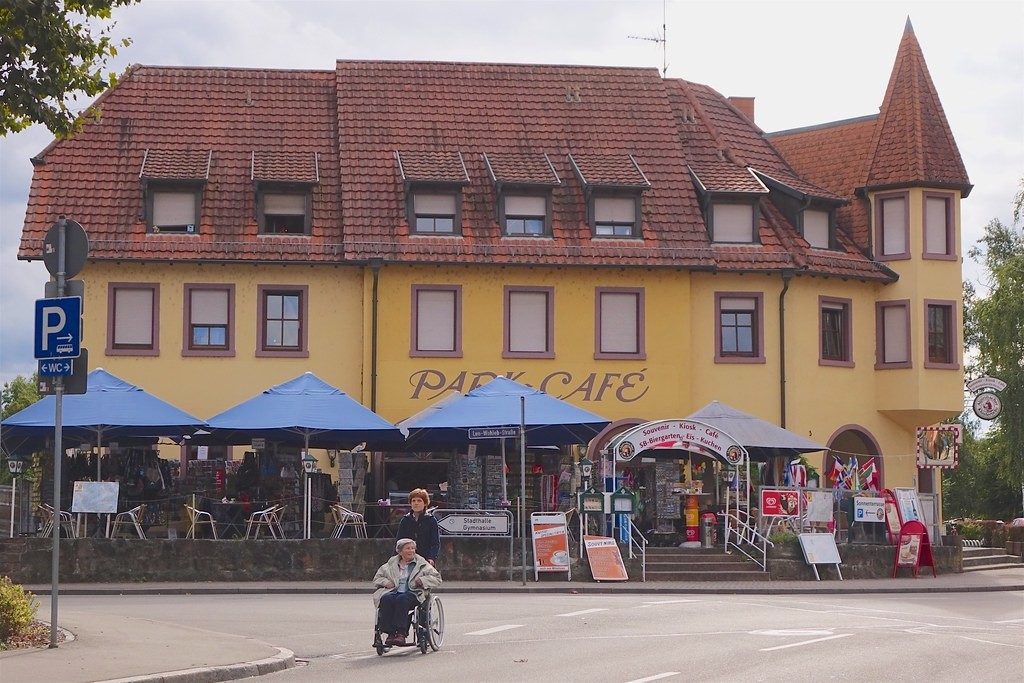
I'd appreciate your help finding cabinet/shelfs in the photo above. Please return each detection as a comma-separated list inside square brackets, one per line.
[506, 449, 541, 534]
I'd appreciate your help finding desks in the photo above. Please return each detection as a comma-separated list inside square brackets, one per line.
[211, 501, 252, 537]
[370, 504, 410, 537]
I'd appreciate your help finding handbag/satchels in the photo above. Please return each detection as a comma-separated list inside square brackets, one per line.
[70, 450, 173, 497]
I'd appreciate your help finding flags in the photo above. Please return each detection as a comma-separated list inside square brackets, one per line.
[827, 455, 879, 500]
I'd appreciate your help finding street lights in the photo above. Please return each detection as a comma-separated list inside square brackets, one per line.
[6, 454, 24, 537]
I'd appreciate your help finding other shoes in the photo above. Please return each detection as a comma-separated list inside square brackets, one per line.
[394, 632, 405, 645]
[417, 642, 429, 646]
[385, 633, 396, 644]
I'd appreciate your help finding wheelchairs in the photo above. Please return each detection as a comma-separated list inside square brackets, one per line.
[372, 561, 445, 656]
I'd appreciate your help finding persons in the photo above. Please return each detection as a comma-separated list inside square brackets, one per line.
[371, 538, 442, 645]
[391, 488, 440, 646]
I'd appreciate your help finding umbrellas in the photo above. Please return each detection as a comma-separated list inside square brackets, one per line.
[406, 375, 613, 509]
[635, 401, 830, 505]
[0, 368, 209, 537]
[205, 372, 400, 540]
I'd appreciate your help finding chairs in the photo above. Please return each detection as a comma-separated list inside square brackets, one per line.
[36, 501, 369, 538]
